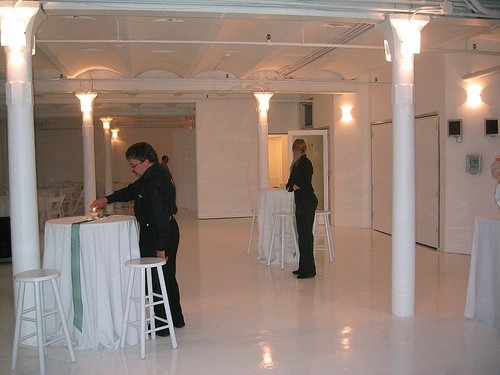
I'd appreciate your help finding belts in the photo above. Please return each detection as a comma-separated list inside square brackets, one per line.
[140, 214, 173, 226]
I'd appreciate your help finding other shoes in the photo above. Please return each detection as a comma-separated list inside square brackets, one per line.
[147, 323, 169, 337]
[173, 321, 185, 328]
[292, 270, 316, 279]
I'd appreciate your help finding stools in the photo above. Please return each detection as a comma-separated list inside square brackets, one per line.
[120, 256, 178, 360]
[267, 213, 299, 270]
[11, 269, 76, 375]
[248, 209, 258, 255]
[313, 210, 335, 263]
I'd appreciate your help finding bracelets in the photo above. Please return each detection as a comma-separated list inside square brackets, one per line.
[104, 197, 108, 202]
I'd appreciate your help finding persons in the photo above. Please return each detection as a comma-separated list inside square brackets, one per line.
[90, 141, 185, 336]
[286, 139, 318, 279]
[161, 155, 170, 171]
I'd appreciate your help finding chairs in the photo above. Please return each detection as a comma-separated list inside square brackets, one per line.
[40, 180, 106, 224]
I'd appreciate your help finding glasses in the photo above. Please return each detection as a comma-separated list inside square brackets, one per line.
[129, 162, 142, 170]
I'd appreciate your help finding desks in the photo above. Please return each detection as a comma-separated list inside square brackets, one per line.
[42, 215, 149, 350]
[258, 188, 298, 264]
[464, 215, 500, 328]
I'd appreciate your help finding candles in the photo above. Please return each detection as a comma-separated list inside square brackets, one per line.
[91, 207, 97, 217]
